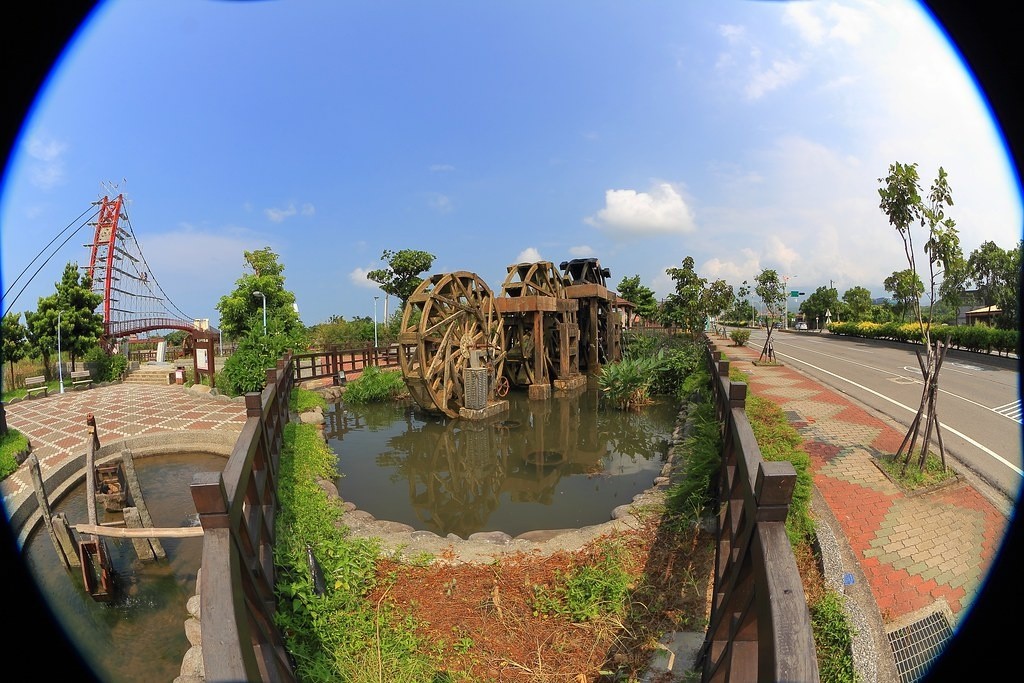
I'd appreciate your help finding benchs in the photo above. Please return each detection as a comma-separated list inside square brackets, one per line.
[70, 370, 93, 391]
[25, 375, 49, 400]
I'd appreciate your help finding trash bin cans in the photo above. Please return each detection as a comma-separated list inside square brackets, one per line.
[174, 366, 187, 385]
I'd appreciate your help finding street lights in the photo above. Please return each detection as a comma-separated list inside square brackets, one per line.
[253, 291, 267, 335]
[58, 309, 66, 394]
[374, 296, 380, 348]
[783, 275, 797, 329]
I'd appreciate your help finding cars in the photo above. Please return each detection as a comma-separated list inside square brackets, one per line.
[795, 322, 808, 330]
[762, 323, 767, 327]
[774, 322, 783, 329]
